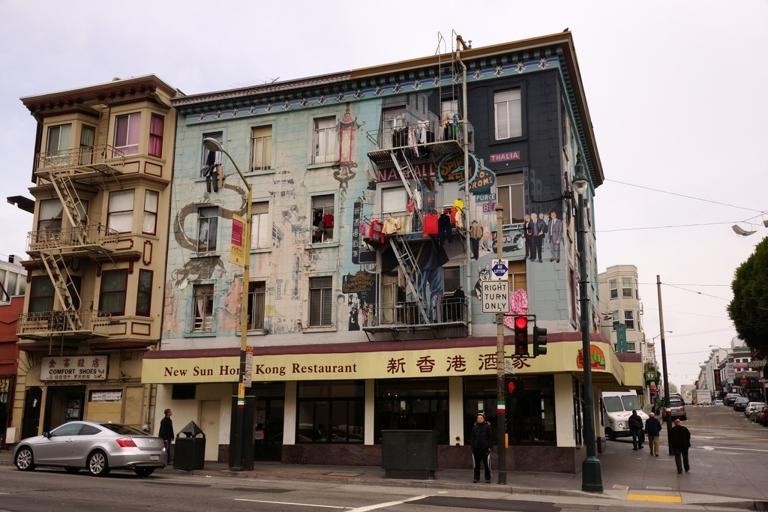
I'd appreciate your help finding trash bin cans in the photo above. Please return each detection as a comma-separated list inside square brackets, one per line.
[173, 420, 206, 470]
[382, 430, 437, 479]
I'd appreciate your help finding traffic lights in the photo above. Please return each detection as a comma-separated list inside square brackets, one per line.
[532, 325, 548, 357]
[504, 376, 524, 396]
[513, 315, 530, 356]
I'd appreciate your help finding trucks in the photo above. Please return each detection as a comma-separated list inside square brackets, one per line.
[600, 390, 650, 440]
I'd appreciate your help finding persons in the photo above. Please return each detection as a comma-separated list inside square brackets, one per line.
[524, 209, 562, 263]
[470, 219, 483, 259]
[465, 414, 495, 484]
[629, 409, 690, 474]
[159, 408, 174, 463]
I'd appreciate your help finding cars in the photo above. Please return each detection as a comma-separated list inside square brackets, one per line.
[745, 399, 768, 424]
[11, 419, 170, 480]
[296, 420, 363, 444]
[661, 388, 751, 423]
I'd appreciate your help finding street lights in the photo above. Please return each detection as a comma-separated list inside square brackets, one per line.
[568, 159, 605, 494]
[706, 343, 730, 378]
[199, 135, 257, 472]
[650, 329, 674, 379]
